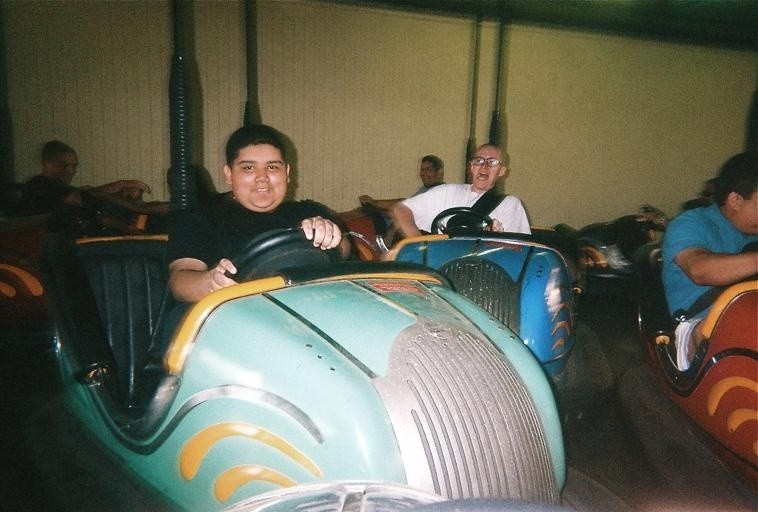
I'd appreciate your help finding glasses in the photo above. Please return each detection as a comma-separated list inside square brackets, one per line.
[469, 156, 502, 167]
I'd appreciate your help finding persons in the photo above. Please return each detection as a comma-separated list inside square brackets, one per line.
[360, 154, 444, 242]
[662, 151, 758, 371]
[73, 163, 216, 236]
[157, 122, 354, 357]
[391, 142, 531, 239]
[1, 138, 152, 215]
